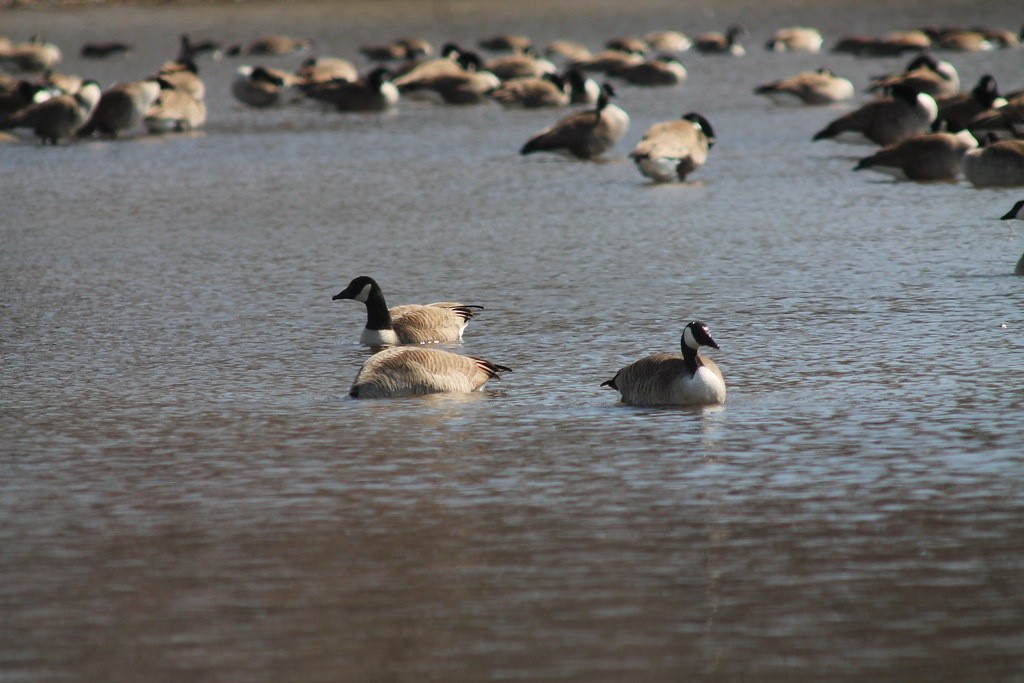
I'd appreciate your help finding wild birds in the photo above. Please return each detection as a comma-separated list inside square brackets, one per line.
[517, 80, 629, 161]
[350, 347, 513, 399]
[598, 321, 726, 404]
[855, 119, 981, 186]
[332, 275, 485, 346]
[631, 108, 713, 181]
[960, 136, 1024, 190]
[1000, 200, 1024, 275]
[0, 23, 1024, 139]
